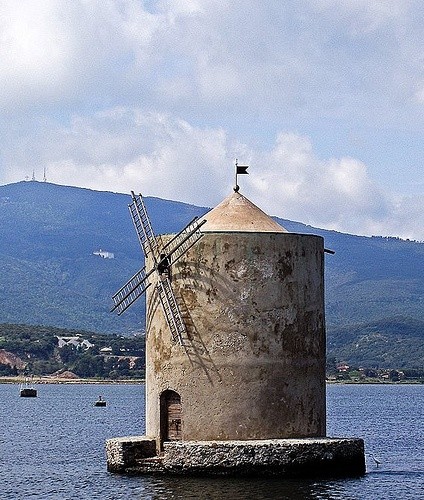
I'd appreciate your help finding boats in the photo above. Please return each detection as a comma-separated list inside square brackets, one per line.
[20, 388, 38, 398]
[94, 401, 106, 407]
